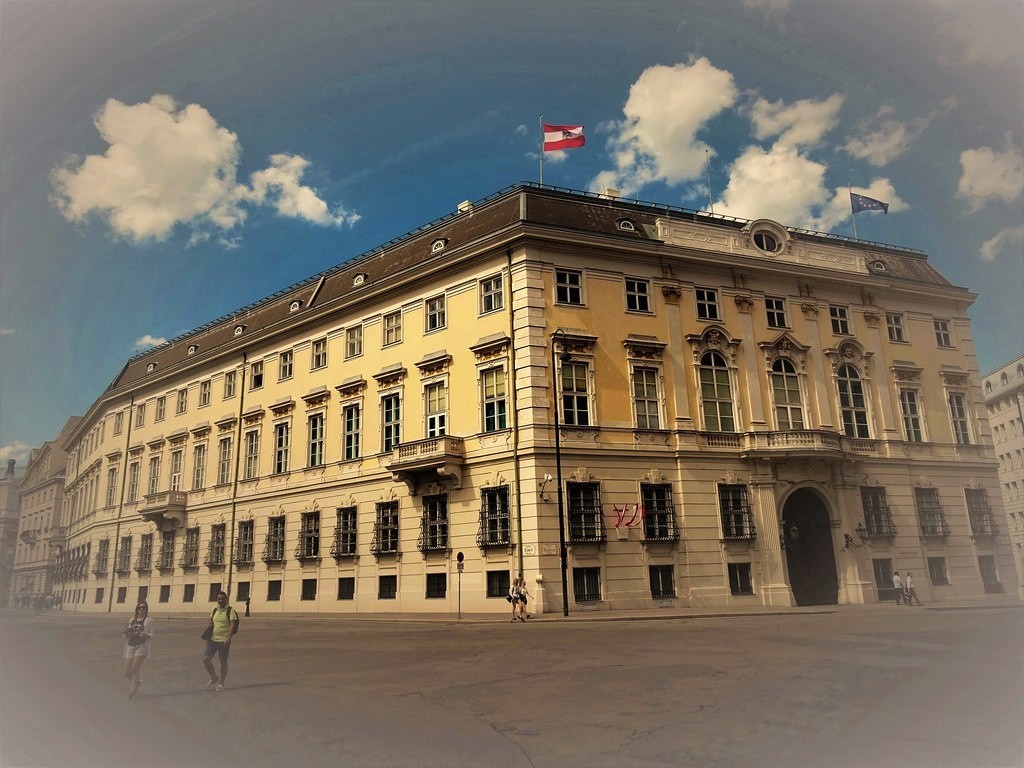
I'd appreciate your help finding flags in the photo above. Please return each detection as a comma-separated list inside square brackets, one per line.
[850, 193, 889, 215]
[540, 122, 585, 152]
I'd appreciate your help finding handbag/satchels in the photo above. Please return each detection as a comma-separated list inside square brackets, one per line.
[201, 608, 217, 641]
[506, 595, 513, 603]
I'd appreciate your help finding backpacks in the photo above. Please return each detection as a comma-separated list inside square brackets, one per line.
[213, 606, 239, 634]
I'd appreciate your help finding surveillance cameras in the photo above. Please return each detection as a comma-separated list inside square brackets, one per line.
[548, 475, 553, 482]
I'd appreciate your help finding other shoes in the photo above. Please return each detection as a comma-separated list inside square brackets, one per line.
[207, 676, 218, 688]
[216, 684, 224, 692]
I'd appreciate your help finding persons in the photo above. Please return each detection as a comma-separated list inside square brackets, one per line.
[201, 590, 239, 692]
[893, 571, 909, 605]
[904, 572, 923, 606]
[12, 593, 64, 613]
[509, 578, 525, 622]
[121, 601, 156, 703]
[517, 581, 534, 619]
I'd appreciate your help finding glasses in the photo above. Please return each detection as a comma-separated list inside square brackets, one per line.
[138, 606, 147, 610]
